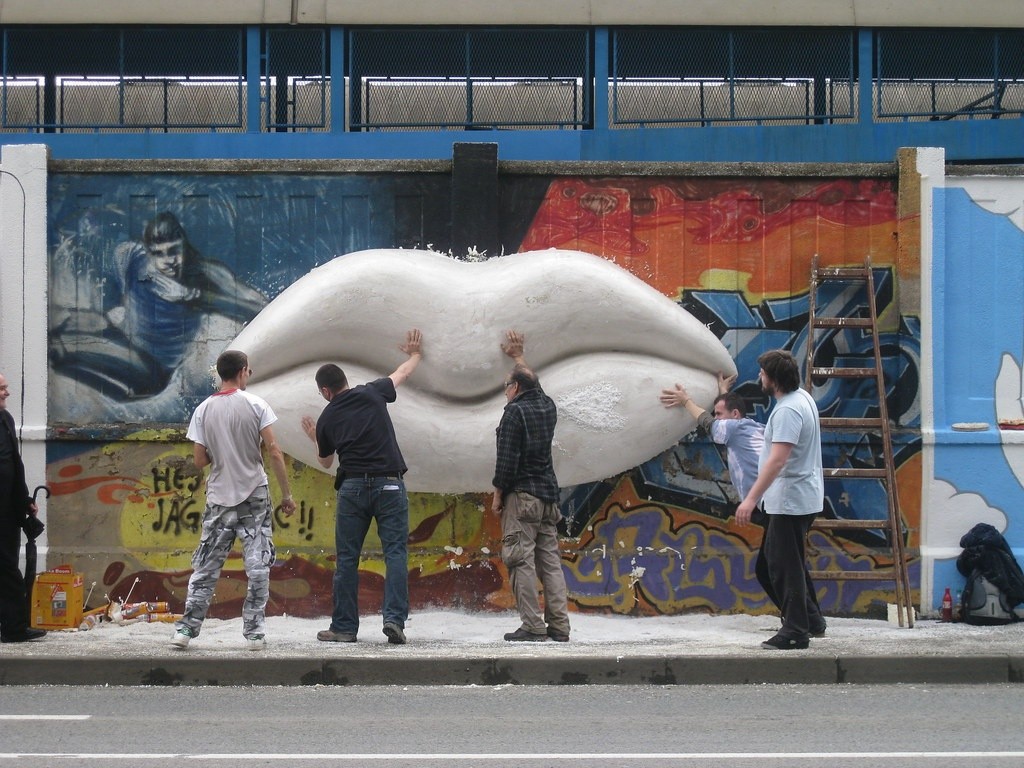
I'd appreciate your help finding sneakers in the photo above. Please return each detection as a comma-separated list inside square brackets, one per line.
[760, 635, 810, 650]
[504, 627, 548, 642]
[549, 632, 568, 641]
[172, 628, 191, 647]
[383, 622, 406, 644]
[317, 628, 357, 642]
[246, 635, 266, 650]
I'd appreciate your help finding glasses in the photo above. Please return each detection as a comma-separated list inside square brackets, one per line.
[245, 368, 253, 376]
[504, 381, 516, 388]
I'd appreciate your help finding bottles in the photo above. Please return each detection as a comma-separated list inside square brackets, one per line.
[79, 600, 184, 631]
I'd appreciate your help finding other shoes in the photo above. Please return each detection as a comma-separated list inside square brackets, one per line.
[810, 629, 825, 638]
[1, 627, 47, 642]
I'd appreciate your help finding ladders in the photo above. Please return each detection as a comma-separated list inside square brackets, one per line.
[804, 254, 913, 629]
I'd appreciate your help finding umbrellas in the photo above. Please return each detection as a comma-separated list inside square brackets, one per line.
[22, 486, 50, 625]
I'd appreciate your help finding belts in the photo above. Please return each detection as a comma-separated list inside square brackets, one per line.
[344, 472, 402, 480]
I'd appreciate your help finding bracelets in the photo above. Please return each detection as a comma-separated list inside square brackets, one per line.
[282, 495, 292, 501]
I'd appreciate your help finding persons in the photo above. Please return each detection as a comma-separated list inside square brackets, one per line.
[169, 349, 295, 649]
[733, 350, 824, 649]
[301, 330, 423, 644]
[491, 328, 571, 643]
[658, 370, 827, 636]
[0, 374, 46, 643]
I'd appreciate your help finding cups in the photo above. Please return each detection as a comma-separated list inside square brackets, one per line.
[942, 588, 952, 620]
